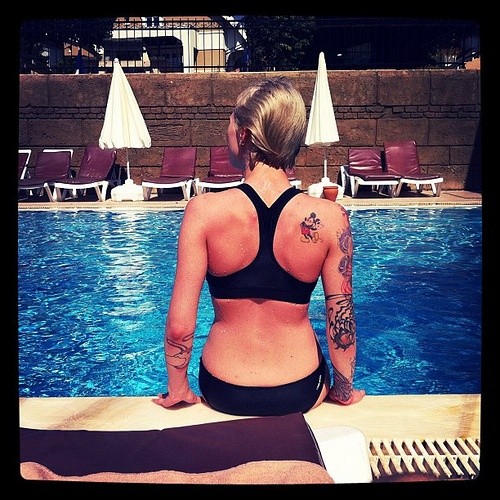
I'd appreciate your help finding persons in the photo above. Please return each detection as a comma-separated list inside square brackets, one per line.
[151, 81, 364, 416]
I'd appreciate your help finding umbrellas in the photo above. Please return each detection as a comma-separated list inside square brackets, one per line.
[305, 52, 340, 177]
[99, 57, 152, 179]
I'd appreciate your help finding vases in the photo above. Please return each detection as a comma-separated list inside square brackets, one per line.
[323, 185, 338, 203]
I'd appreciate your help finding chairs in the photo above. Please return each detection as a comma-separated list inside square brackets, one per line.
[21, 409, 332, 485]
[142, 146, 200, 203]
[17, 145, 118, 203]
[285, 164, 301, 190]
[379, 139, 443, 198]
[342, 147, 402, 199]
[198, 144, 244, 194]
